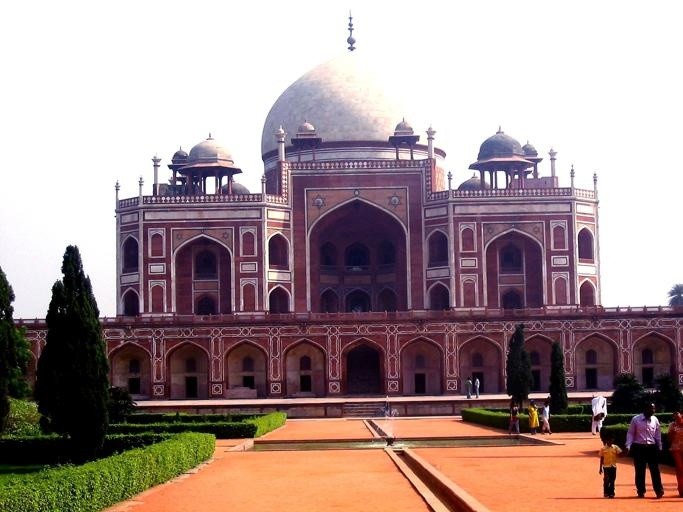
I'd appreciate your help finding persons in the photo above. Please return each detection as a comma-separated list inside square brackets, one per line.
[591, 396, 607, 435]
[465, 377, 472, 399]
[625, 402, 664, 499]
[667, 410, 683, 497]
[528, 400, 539, 435]
[509, 401, 520, 434]
[474, 377, 480, 398]
[599, 434, 623, 498]
[541, 402, 552, 434]
